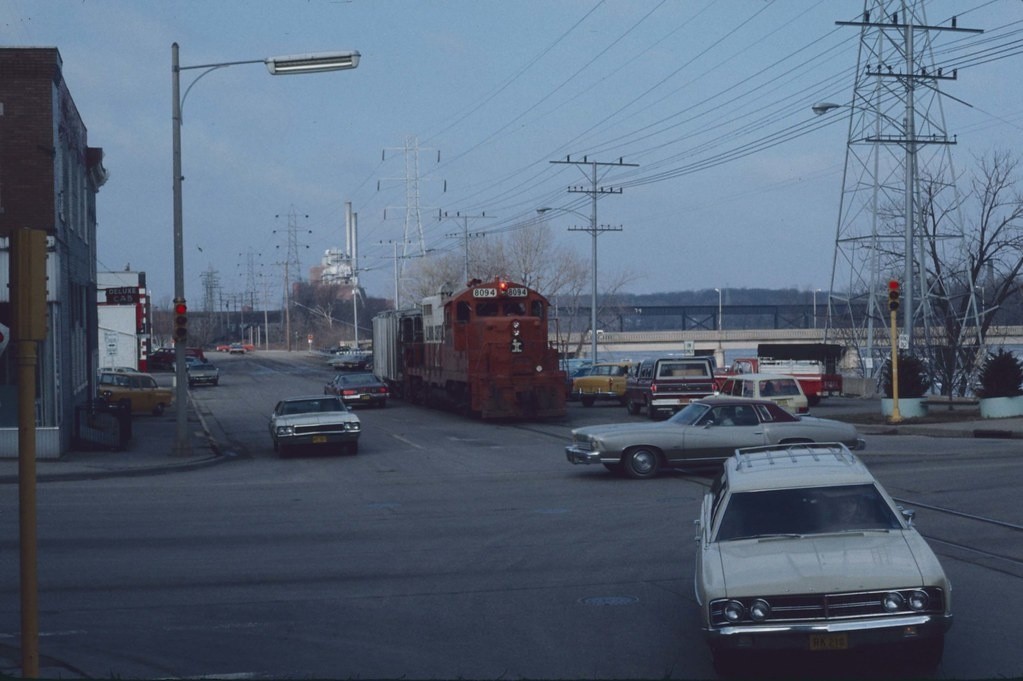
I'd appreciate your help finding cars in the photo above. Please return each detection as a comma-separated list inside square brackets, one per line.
[706, 374, 811, 418]
[149, 347, 219, 385]
[693, 441, 953, 673]
[216, 341, 255, 354]
[327, 346, 374, 371]
[323, 372, 390, 407]
[560, 356, 633, 420]
[268, 394, 362, 456]
[96, 367, 173, 416]
[566, 395, 868, 479]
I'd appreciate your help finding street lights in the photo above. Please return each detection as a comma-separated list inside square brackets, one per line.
[975, 285, 985, 325]
[813, 289, 822, 328]
[169, 42, 361, 460]
[536, 206, 597, 367]
[715, 288, 722, 349]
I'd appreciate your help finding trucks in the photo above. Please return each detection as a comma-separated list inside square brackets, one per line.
[712, 358, 844, 406]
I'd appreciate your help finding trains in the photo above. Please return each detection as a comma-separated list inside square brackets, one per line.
[371, 273, 570, 422]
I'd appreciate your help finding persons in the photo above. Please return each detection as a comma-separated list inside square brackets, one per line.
[720, 408, 735, 426]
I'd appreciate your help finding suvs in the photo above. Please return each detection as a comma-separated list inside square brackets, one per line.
[625, 356, 718, 420]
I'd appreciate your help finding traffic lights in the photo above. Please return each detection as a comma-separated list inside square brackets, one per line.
[174, 300, 189, 341]
[888, 279, 900, 311]
